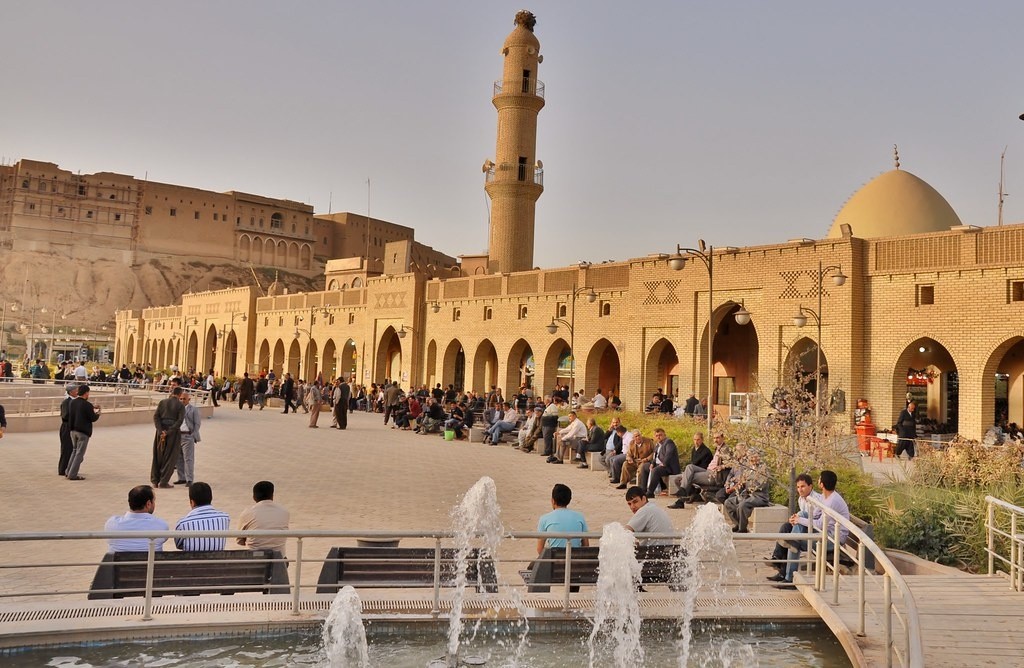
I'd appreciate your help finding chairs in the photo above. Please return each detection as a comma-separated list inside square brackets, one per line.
[869, 437, 894, 462]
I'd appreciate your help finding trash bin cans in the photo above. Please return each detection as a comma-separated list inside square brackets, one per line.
[854, 422, 876, 450]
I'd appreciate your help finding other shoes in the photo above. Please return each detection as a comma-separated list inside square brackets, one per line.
[660, 484, 667, 490]
[152, 483, 158, 488]
[71, 476, 85, 480]
[383, 422, 435, 436]
[541, 454, 550, 456]
[731, 524, 739, 532]
[668, 503, 685, 509]
[573, 457, 582, 462]
[482, 431, 531, 453]
[331, 426, 337, 428]
[185, 481, 193, 487]
[158, 483, 173, 488]
[673, 488, 687, 498]
[767, 574, 786, 581]
[576, 463, 589, 468]
[763, 557, 780, 569]
[173, 480, 187, 485]
[645, 492, 655, 498]
[616, 483, 627, 489]
[551, 460, 562, 464]
[777, 538, 800, 553]
[611, 478, 621, 484]
[772, 580, 797, 590]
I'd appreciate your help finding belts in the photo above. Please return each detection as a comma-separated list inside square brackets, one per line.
[179, 431, 191, 434]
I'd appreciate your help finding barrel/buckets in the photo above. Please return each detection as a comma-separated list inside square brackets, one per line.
[444, 430, 454, 441]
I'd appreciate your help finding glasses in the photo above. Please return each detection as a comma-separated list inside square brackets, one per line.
[179, 397, 187, 400]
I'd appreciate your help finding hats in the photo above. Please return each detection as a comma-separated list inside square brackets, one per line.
[65, 386, 78, 390]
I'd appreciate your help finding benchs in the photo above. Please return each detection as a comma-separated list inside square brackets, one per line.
[391, 408, 789, 533]
[214, 391, 282, 407]
[518, 545, 692, 593]
[316, 548, 499, 593]
[87, 548, 273, 600]
[812, 513, 875, 571]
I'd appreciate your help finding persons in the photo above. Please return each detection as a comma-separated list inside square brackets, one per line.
[0, 404, 7, 438]
[0, 350, 13, 382]
[150, 365, 787, 533]
[762, 470, 850, 590]
[620, 486, 673, 546]
[22, 353, 154, 389]
[174, 482, 230, 551]
[104, 485, 168, 551]
[535, 484, 590, 592]
[58, 384, 101, 480]
[235, 481, 290, 558]
[895, 393, 918, 460]
